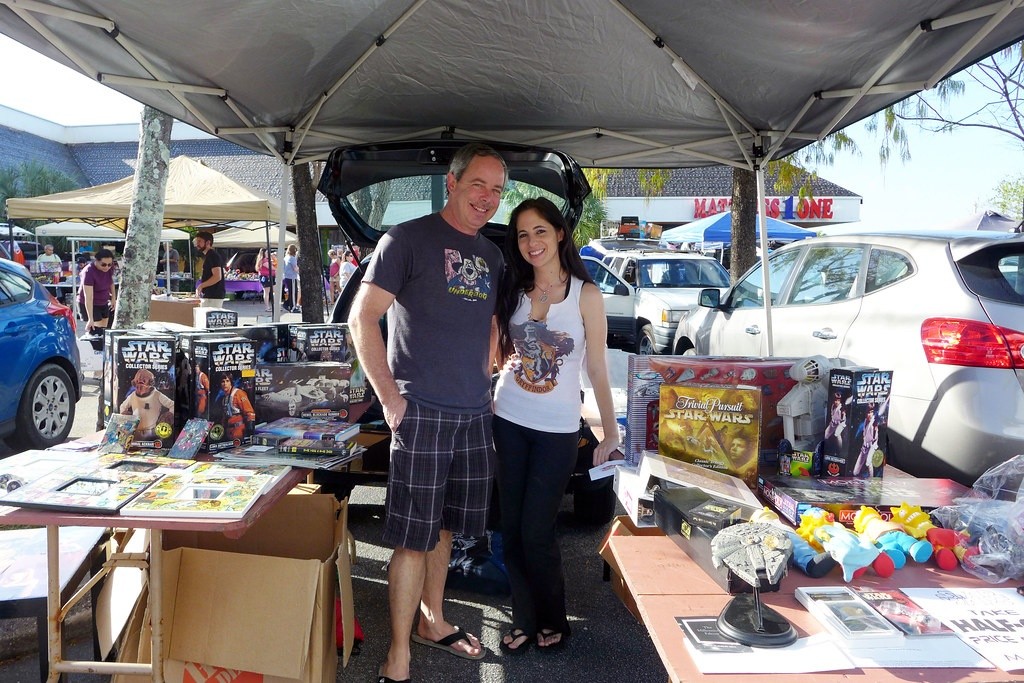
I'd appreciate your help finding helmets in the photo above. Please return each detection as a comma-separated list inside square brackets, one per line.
[131, 370, 155, 390]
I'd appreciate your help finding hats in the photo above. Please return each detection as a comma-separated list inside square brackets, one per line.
[77, 257, 85, 263]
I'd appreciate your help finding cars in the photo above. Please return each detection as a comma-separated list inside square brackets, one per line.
[0, 256, 84, 450]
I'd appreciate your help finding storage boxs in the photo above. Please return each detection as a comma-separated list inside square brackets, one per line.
[102, 300, 366, 451]
[96, 491, 353, 683]
[613, 352, 987, 598]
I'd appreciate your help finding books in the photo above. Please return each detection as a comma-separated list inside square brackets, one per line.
[250, 417, 361, 458]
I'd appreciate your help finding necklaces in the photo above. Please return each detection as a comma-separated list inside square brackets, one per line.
[535, 282, 548, 303]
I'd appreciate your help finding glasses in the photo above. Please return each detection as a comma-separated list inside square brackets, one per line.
[350, 255, 353, 257]
[100, 261, 112, 267]
[136, 386, 147, 391]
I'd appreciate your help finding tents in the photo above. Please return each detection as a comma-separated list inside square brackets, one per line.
[35, 222, 193, 292]
[660, 210, 817, 265]
[193, 221, 299, 248]
[6, 156, 297, 322]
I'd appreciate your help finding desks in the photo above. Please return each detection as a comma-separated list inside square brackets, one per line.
[41, 283, 118, 298]
[607, 463, 1024, 683]
[0, 389, 375, 682]
[196, 278, 263, 304]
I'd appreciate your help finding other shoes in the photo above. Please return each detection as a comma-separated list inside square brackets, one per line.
[266, 309, 272, 312]
[291, 308, 300, 313]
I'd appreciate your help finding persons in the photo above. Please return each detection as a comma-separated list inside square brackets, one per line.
[194, 360, 211, 418]
[255, 243, 360, 312]
[287, 337, 301, 363]
[195, 232, 226, 308]
[160, 241, 180, 292]
[119, 369, 174, 442]
[37, 244, 62, 298]
[716, 424, 756, 464]
[214, 372, 256, 443]
[347, 145, 508, 683]
[824, 391, 846, 456]
[76, 241, 116, 331]
[853, 402, 878, 478]
[491, 196, 619, 657]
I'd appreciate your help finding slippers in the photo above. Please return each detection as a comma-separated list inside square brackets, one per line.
[536, 627, 564, 650]
[378, 664, 411, 683]
[500, 628, 533, 655]
[412, 626, 487, 660]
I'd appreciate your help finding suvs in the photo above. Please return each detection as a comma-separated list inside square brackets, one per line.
[579, 237, 731, 356]
[0, 222, 45, 268]
[314, 136, 593, 507]
[671, 229, 1024, 485]
